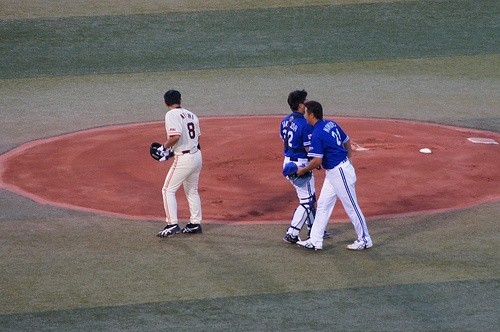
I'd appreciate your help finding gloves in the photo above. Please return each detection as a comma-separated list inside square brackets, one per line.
[156, 144, 165, 156]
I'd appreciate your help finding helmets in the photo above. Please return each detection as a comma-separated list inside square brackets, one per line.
[150, 142, 174, 162]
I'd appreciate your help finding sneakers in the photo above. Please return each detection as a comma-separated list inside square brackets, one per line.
[296, 239, 323, 251]
[176, 222, 202, 234]
[347, 240, 374, 250]
[156, 224, 181, 238]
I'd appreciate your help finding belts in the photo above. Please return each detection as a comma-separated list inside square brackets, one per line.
[290, 157, 310, 162]
[343, 158, 347, 163]
[182, 144, 200, 154]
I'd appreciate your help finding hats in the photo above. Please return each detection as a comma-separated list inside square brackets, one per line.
[283, 162, 298, 177]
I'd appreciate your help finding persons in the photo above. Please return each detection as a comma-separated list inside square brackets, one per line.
[279, 89, 333, 244]
[150, 90, 202, 237]
[296, 101, 373, 252]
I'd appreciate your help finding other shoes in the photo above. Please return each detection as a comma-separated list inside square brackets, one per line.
[282, 232, 303, 244]
[307, 230, 332, 239]
